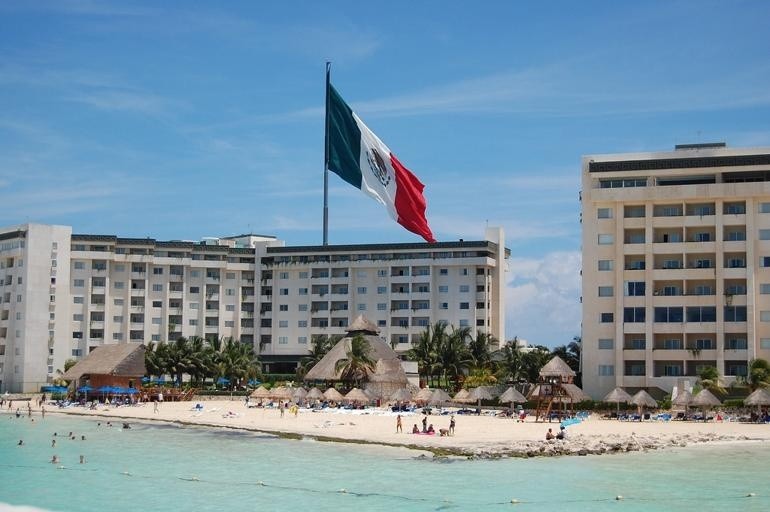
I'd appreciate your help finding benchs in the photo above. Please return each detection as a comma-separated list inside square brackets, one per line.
[326, 84, 435, 242]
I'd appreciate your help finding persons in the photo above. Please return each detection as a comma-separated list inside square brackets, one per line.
[556, 425, 567, 440]
[279, 400, 286, 418]
[19, 438, 25, 445]
[80, 455, 86, 464]
[0, 397, 36, 422]
[690, 388, 723, 423]
[159, 390, 165, 400]
[669, 390, 694, 418]
[53, 399, 125, 410]
[152, 400, 160, 412]
[98, 420, 131, 430]
[38, 393, 47, 405]
[603, 387, 631, 416]
[396, 414, 404, 433]
[51, 430, 87, 447]
[50, 454, 60, 463]
[41, 406, 46, 420]
[630, 389, 658, 415]
[294, 403, 301, 418]
[545, 427, 555, 440]
[412, 412, 455, 436]
[743, 387, 770, 420]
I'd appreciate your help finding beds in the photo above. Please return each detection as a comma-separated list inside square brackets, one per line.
[77, 383, 140, 401]
[248, 384, 528, 413]
[46, 386, 71, 395]
